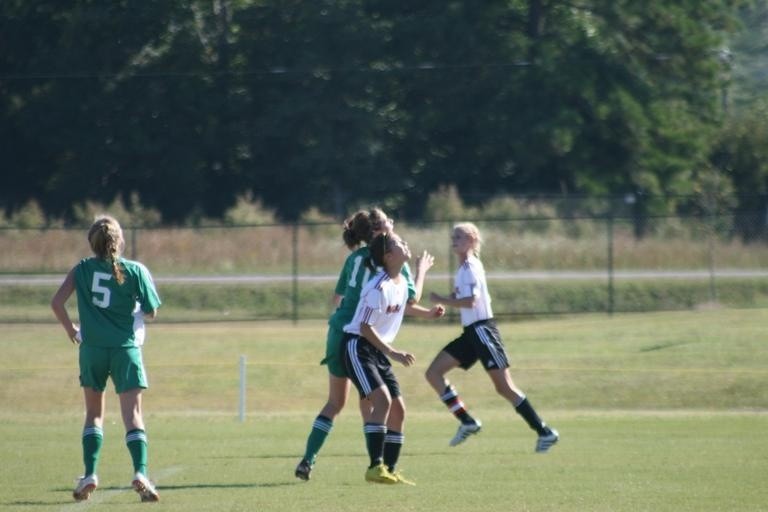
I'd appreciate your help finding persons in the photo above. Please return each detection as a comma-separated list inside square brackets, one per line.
[295, 208, 435, 480]
[339, 230, 445, 487]
[51, 215, 162, 502]
[425, 222, 559, 453]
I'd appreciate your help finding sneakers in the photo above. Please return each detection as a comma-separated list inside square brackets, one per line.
[536, 432, 559, 452]
[366, 464, 414, 484]
[296, 462, 310, 481]
[450, 422, 482, 446]
[133, 472, 159, 502]
[73, 475, 98, 501]
